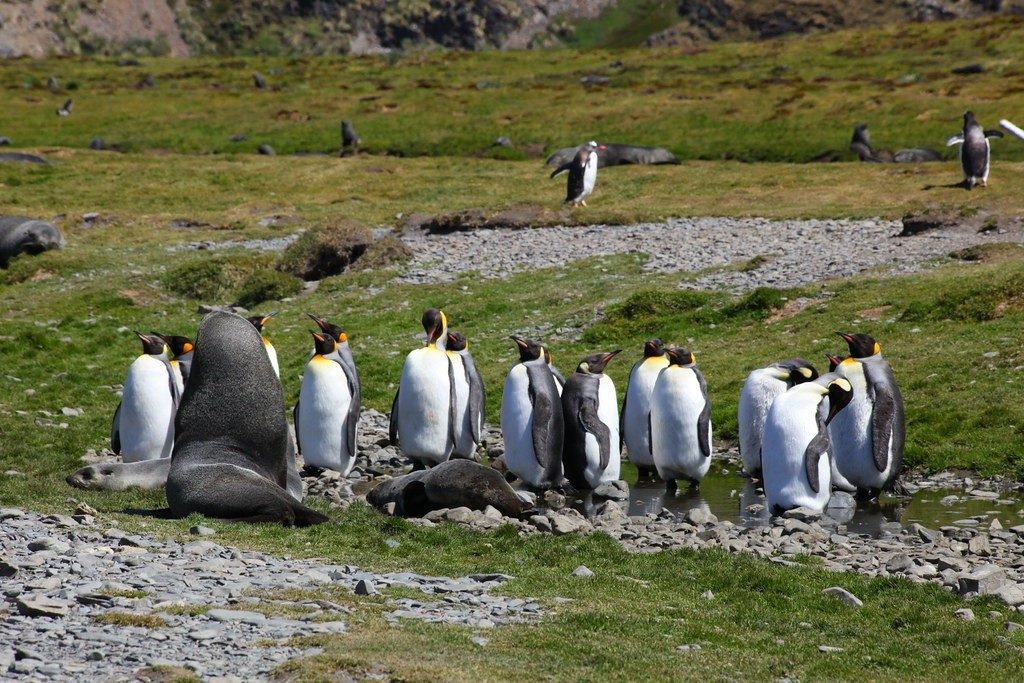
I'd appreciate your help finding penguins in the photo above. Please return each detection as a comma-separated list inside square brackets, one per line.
[110, 330, 181, 463]
[550, 139, 607, 208]
[293, 328, 361, 479]
[830, 331, 913, 503]
[737, 358, 820, 480]
[444, 330, 486, 463]
[243, 311, 281, 379]
[151, 331, 195, 404]
[759, 372, 854, 516]
[646, 346, 713, 490]
[619, 338, 671, 483]
[825, 352, 859, 491]
[945, 111, 1005, 190]
[307, 313, 358, 381]
[389, 308, 461, 474]
[561, 349, 622, 491]
[498, 335, 564, 495]
